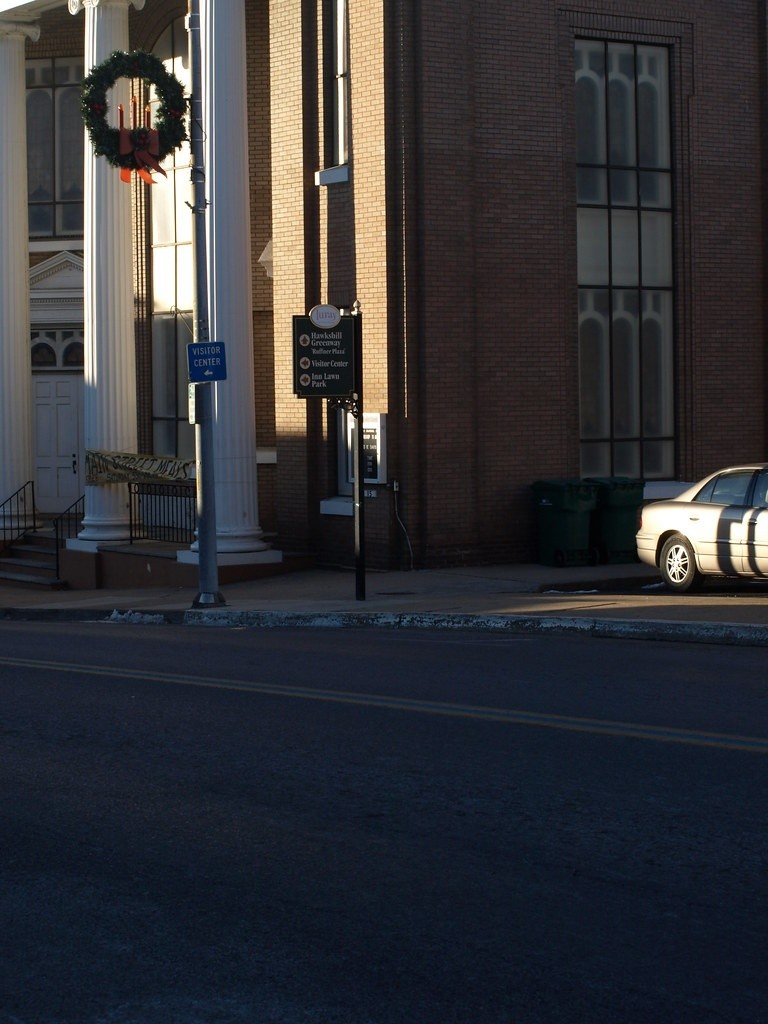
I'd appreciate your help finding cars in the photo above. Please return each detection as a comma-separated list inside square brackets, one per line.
[635, 463, 768, 593]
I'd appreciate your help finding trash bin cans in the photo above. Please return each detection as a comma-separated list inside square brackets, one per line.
[529, 476, 599, 567]
[582, 477, 645, 565]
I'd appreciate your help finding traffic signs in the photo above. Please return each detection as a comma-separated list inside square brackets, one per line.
[292, 304, 355, 397]
[185, 343, 227, 383]
